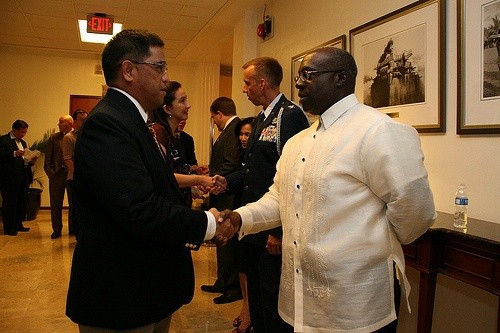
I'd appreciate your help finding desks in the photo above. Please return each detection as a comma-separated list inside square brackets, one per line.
[401, 210, 500, 333]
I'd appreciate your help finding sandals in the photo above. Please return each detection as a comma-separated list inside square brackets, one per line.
[232, 322, 253, 333]
[233, 317, 242, 327]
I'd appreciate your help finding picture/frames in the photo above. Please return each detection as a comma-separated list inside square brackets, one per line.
[348, 0, 447, 133]
[290, 34, 346, 124]
[456, 0, 500, 137]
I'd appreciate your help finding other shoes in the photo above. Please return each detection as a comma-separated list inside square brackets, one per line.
[16, 224, 30, 232]
[5, 228, 17, 236]
[69, 230, 73, 234]
[51, 231, 62, 239]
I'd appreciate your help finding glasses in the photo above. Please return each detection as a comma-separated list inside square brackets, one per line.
[295, 70, 345, 83]
[118, 61, 167, 73]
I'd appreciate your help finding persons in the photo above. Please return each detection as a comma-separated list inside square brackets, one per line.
[214, 47, 438, 333]
[61, 108, 87, 239]
[43, 115, 78, 240]
[152, 80, 226, 210]
[201, 96, 257, 333]
[0, 120, 37, 236]
[205, 58, 309, 333]
[63, 29, 212, 318]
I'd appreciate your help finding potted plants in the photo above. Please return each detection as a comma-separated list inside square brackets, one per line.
[25, 127, 56, 222]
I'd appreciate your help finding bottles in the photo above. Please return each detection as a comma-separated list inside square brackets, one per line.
[453, 184, 469, 229]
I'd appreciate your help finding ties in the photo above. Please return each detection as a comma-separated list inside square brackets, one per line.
[253, 113, 266, 141]
[148, 118, 161, 158]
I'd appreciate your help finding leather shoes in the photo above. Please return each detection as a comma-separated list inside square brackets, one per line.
[214, 293, 243, 304]
[201, 285, 219, 292]
